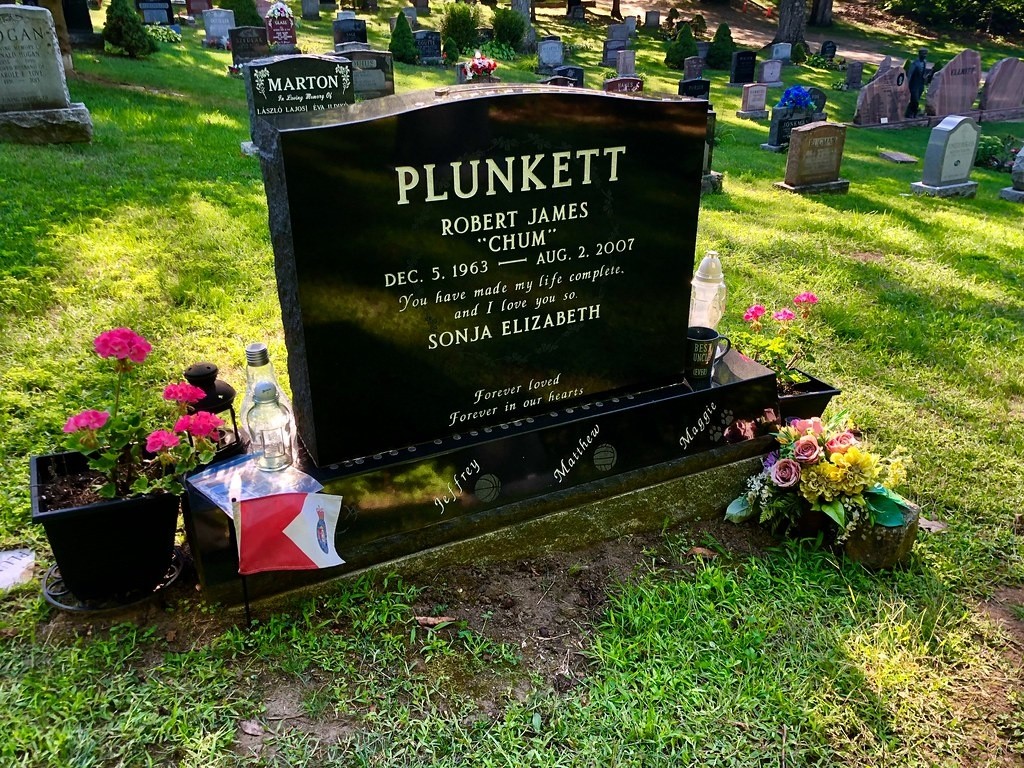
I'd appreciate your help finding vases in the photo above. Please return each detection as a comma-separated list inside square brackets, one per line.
[778, 368, 843, 426]
[30, 443, 186, 603]
[779, 495, 840, 546]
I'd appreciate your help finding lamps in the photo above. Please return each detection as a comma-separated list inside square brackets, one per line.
[184, 362, 240, 470]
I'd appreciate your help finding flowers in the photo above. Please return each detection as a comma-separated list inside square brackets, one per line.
[62, 328, 225, 497]
[265, 2, 294, 28]
[776, 84, 818, 115]
[730, 292, 830, 394]
[724, 407, 915, 546]
[462, 51, 498, 80]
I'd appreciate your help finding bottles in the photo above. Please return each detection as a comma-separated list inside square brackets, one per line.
[689, 252, 726, 328]
[240, 343, 298, 462]
[248, 382, 294, 472]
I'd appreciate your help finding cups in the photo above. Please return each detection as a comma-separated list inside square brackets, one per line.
[687, 327, 732, 381]
